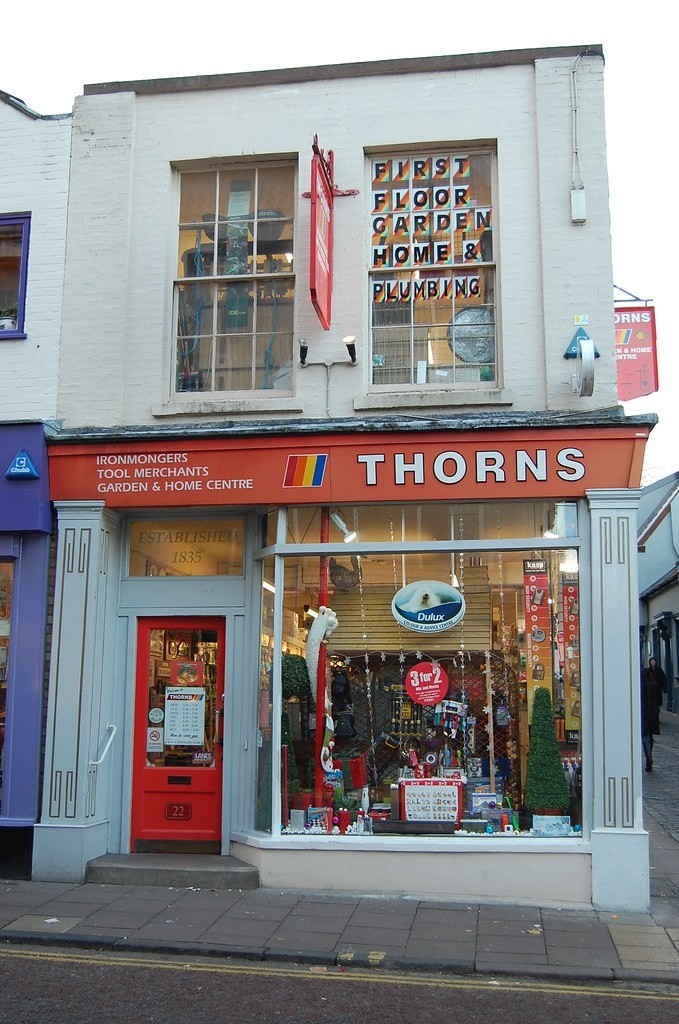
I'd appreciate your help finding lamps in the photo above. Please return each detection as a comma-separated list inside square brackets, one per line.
[449, 505, 460, 588]
[330, 511, 357, 543]
[343, 335, 356, 363]
[543, 515, 562, 538]
[298, 338, 310, 365]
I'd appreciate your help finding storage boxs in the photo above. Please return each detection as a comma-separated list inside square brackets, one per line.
[398, 776, 464, 821]
[466, 792, 497, 812]
[338, 810, 365, 834]
[293, 792, 314, 811]
[427, 361, 481, 384]
[325, 771, 344, 792]
[373, 321, 427, 384]
[333, 752, 370, 789]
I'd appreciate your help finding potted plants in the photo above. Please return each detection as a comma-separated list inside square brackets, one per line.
[0, 297, 17, 330]
[524, 685, 570, 816]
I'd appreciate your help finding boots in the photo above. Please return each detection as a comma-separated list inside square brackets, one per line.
[642, 738, 654, 772]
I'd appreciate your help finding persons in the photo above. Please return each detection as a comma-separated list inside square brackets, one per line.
[640, 657, 667, 772]
